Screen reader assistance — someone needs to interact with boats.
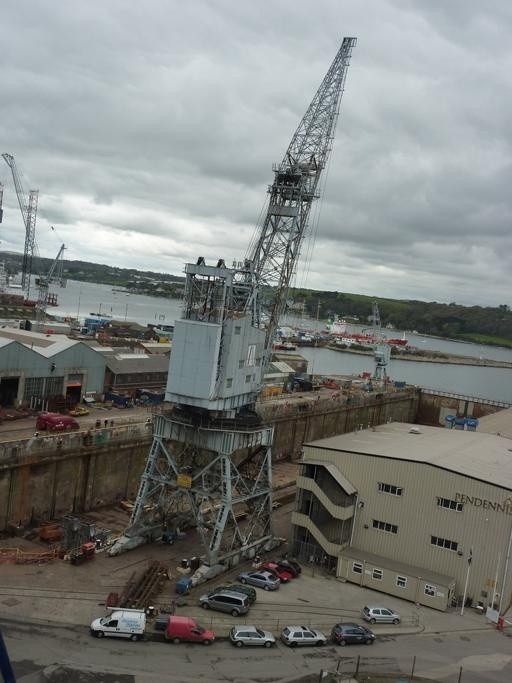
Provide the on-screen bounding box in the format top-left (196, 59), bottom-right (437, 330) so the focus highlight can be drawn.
top-left (261, 297), bottom-right (409, 354)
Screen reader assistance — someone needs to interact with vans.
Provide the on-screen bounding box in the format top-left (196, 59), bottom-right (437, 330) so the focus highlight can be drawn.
top-left (91, 606), bottom-right (147, 642)
top-left (164, 615), bottom-right (216, 646)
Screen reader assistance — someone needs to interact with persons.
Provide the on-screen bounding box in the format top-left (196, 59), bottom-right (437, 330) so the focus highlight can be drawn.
top-left (145, 415), bottom-right (152, 432)
top-left (111, 419), bottom-right (114, 427)
top-left (104, 418), bottom-right (109, 428)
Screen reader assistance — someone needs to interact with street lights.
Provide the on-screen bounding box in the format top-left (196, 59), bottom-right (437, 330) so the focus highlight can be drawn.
top-left (459, 547), bottom-right (472, 615)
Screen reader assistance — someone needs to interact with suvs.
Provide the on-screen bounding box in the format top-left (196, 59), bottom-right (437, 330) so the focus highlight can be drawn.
top-left (331, 622), bottom-right (375, 645)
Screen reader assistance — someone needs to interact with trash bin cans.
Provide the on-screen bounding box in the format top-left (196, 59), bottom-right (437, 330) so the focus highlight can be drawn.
top-left (81, 543), bottom-right (96, 561)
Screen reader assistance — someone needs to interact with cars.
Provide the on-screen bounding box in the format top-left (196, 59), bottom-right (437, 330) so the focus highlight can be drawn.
top-left (199, 556), bottom-right (302, 616)
top-left (1, 403), bottom-right (90, 433)
top-left (280, 624), bottom-right (328, 647)
top-left (155, 612), bottom-right (170, 629)
top-left (230, 625), bottom-right (276, 648)
top-left (363, 604), bottom-right (400, 625)
